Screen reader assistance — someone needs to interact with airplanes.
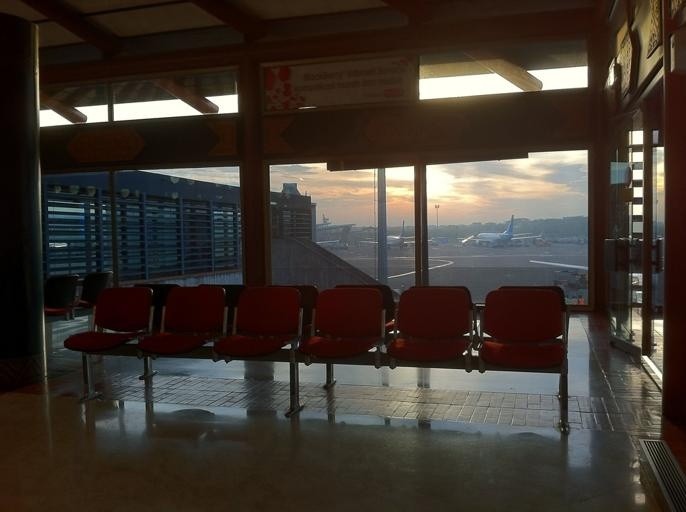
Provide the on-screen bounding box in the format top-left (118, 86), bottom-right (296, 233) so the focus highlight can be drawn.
top-left (456, 214), bottom-right (543, 247)
top-left (359, 218), bottom-right (433, 249)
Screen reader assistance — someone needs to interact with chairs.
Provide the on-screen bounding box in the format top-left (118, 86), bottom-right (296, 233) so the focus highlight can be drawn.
top-left (43, 271), bottom-right (570, 435)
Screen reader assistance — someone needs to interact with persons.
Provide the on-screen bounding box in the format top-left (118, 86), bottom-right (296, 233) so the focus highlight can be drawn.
top-left (569, 296), bottom-right (577, 305)
top-left (564, 294), bottom-right (569, 305)
top-left (577, 295), bottom-right (584, 304)
